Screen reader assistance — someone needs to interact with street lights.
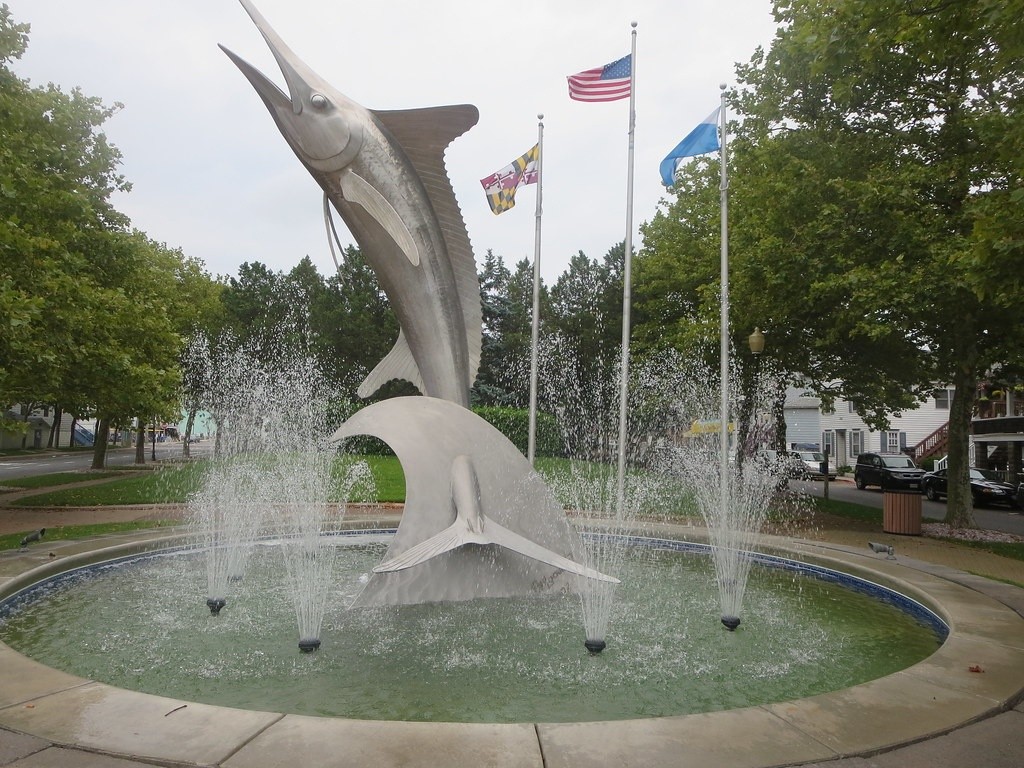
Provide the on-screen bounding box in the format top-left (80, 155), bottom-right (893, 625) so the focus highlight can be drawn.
top-left (748, 325), bottom-right (765, 461)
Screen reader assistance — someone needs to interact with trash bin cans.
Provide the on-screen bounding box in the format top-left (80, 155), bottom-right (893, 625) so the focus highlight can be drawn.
top-left (881, 471), bottom-right (925, 536)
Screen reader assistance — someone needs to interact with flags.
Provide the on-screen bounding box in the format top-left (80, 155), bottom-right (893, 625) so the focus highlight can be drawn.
top-left (659, 106), bottom-right (722, 186)
top-left (565, 53), bottom-right (631, 103)
top-left (480, 143), bottom-right (538, 215)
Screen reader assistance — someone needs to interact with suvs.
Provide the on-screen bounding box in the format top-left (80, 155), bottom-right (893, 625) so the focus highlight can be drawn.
top-left (854, 451), bottom-right (927, 493)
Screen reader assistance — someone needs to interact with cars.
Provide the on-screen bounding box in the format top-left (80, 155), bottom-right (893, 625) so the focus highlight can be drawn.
top-left (751, 450), bottom-right (838, 481)
top-left (160, 437), bottom-right (172, 442)
top-left (921, 466), bottom-right (1016, 507)
top-left (187, 433), bottom-right (200, 444)
